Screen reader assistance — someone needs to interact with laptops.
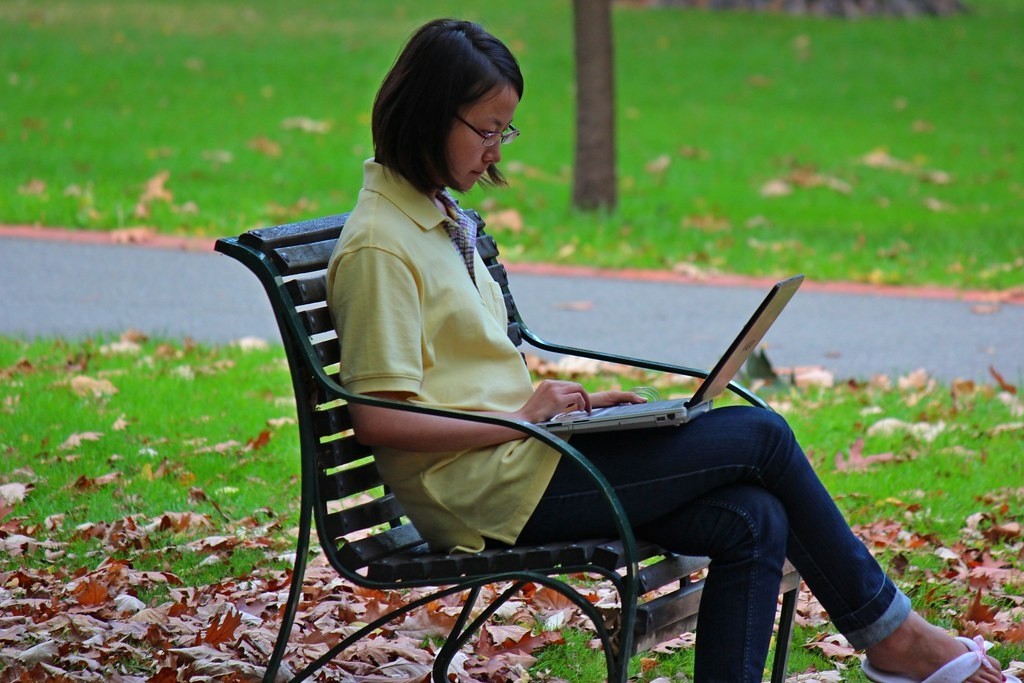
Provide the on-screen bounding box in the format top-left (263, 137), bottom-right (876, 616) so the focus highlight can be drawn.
top-left (533, 273), bottom-right (806, 432)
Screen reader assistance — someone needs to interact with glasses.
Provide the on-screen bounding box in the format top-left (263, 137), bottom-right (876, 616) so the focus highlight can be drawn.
top-left (457, 114), bottom-right (519, 147)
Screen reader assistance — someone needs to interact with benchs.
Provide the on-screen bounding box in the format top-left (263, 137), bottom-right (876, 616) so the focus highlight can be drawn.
top-left (211, 206), bottom-right (802, 683)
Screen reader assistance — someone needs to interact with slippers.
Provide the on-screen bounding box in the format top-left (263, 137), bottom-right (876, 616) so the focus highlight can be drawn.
top-left (861, 634), bottom-right (1022, 683)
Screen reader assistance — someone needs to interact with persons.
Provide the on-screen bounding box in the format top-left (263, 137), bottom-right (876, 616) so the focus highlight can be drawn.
top-left (326, 19), bottom-right (1022, 683)
